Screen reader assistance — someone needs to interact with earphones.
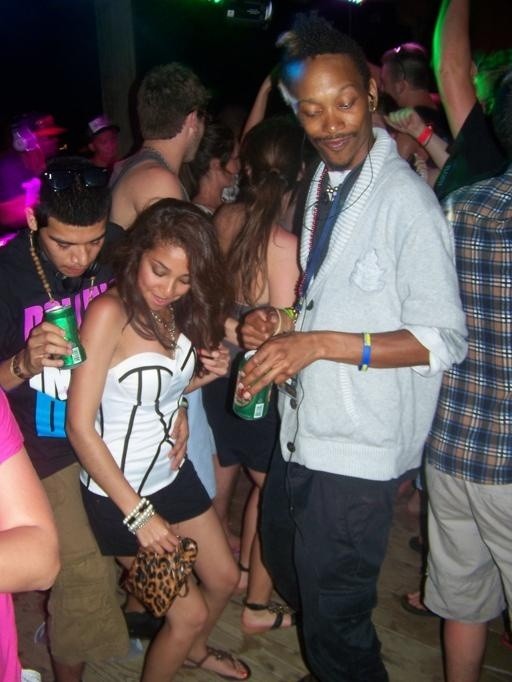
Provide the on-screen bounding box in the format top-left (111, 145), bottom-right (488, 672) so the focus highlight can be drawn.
top-left (365, 94), bottom-right (375, 107)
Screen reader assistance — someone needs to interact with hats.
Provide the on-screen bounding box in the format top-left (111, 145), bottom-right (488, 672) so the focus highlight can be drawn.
top-left (81, 114), bottom-right (120, 146)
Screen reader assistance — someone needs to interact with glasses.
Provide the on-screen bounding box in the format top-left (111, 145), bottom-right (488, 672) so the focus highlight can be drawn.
top-left (392, 45), bottom-right (406, 81)
top-left (40, 167), bottom-right (111, 192)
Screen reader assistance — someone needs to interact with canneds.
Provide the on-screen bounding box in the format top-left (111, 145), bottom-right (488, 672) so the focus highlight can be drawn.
top-left (230, 349), bottom-right (271, 421)
top-left (41, 304), bottom-right (87, 371)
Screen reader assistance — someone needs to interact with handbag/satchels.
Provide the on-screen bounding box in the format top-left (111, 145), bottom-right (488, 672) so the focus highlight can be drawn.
top-left (122, 532), bottom-right (198, 618)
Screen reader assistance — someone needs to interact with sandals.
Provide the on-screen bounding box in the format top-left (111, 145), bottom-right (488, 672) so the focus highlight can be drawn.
top-left (233, 563), bottom-right (249, 597)
top-left (181, 645), bottom-right (250, 681)
top-left (239, 598), bottom-right (296, 635)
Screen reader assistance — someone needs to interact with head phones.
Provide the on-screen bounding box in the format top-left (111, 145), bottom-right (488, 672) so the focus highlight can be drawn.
top-left (12, 126), bottom-right (39, 152)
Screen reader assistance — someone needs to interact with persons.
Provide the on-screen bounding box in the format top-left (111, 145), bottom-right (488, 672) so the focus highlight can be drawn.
top-left (0, 1), bottom-right (511, 633)
top-left (0, 159), bottom-right (189, 681)
top-left (66, 194), bottom-right (251, 682)
top-left (418, 159), bottom-right (512, 682)
top-left (236, 32), bottom-right (470, 682)
top-left (0, 386), bottom-right (63, 681)
